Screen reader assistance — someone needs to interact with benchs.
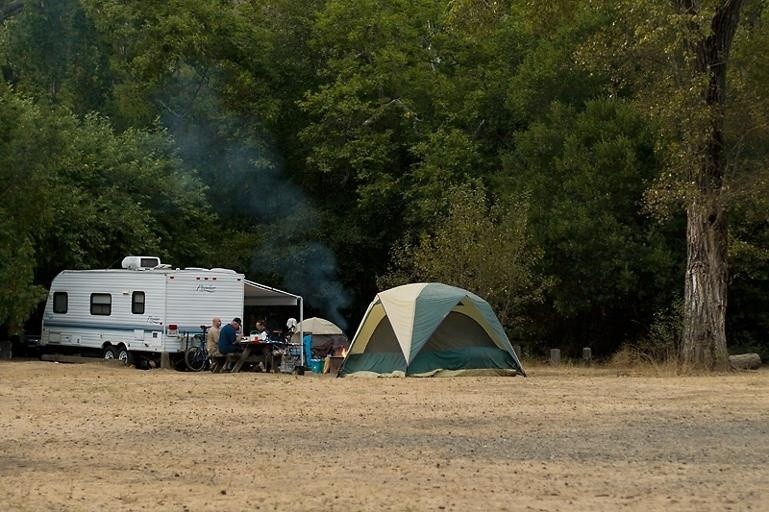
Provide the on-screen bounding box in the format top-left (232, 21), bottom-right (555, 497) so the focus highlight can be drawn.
top-left (207, 351), bottom-right (241, 373)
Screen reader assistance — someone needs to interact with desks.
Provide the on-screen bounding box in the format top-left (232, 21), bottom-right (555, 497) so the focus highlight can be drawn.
top-left (231, 340), bottom-right (301, 373)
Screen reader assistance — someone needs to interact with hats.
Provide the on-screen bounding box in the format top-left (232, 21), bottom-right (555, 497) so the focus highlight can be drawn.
top-left (233, 318), bottom-right (242, 327)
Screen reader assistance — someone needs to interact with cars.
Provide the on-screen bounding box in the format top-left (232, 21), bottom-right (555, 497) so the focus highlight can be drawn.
top-left (12, 329), bottom-right (41, 355)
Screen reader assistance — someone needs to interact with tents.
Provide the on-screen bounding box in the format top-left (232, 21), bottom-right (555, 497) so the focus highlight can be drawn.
top-left (336, 283), bottom-right (526, 378)
top-left (290, 317), bottom-right (350, 356)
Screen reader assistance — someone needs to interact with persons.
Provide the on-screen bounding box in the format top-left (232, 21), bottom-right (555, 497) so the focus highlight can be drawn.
top-left (255, 320), bottom-right (283, 341)
top-left (207, 318), bottom-right (244, 372)
top-left (286, 315), bottom-right (297, 330)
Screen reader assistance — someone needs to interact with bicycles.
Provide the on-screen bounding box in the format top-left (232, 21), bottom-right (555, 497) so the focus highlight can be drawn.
top-left (185, 336), bottom-right (213, 372)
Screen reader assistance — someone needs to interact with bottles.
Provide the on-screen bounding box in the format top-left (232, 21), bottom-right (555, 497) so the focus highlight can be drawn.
top-left (255, 333), bottom-right (258, 341)
